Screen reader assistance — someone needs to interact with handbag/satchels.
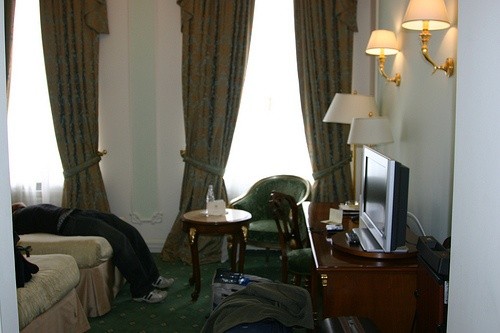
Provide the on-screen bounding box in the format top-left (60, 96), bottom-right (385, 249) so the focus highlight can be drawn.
top-left (210, 275), bottom-right (269, 316)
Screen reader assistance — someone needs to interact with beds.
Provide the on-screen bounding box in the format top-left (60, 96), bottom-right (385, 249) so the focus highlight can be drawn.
top-left (13, 233), bottom-right (128, 333)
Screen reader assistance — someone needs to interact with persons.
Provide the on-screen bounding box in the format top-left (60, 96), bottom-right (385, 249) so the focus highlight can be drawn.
top-left (11, 202), bottom-right (175, 303)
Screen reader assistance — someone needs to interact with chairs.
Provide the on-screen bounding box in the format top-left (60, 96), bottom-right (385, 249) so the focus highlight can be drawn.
top-left (269, 190), bottom-right (319, 321)
top-left (230, 176), bottom-right (307, 274)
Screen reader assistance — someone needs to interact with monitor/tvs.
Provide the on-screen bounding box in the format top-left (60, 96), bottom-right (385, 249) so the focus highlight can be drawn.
top-left (352, 145), bottom-right (410, 253)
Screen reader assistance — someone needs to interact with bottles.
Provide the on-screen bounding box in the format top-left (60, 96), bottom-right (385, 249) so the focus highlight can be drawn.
top-left (206, 185), bottom-right (215, 202)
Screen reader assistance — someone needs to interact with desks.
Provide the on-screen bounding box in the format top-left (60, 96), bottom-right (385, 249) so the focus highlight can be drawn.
top-left (180, 208), bottom-right (252, 301)
top-left (301, 201), bottom-right (424, 333)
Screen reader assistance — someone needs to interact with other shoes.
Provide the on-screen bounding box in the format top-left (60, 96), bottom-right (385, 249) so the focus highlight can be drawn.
top-left (153, 276), bottom-right (174, 288)
top-left (133, 290), bottom-right (167, 303)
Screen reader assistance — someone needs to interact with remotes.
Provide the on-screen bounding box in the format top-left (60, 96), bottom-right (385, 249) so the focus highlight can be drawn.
top-left (346, 232), bottom-right (359, 243)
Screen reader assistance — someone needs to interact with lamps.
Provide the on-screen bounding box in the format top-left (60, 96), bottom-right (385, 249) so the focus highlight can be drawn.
top-left (365, 30), bottom-right (401, 86)
top-left (401, 0), bottom-right (454, 78)
top-left (322, 90), bottom-right (394, 202)
